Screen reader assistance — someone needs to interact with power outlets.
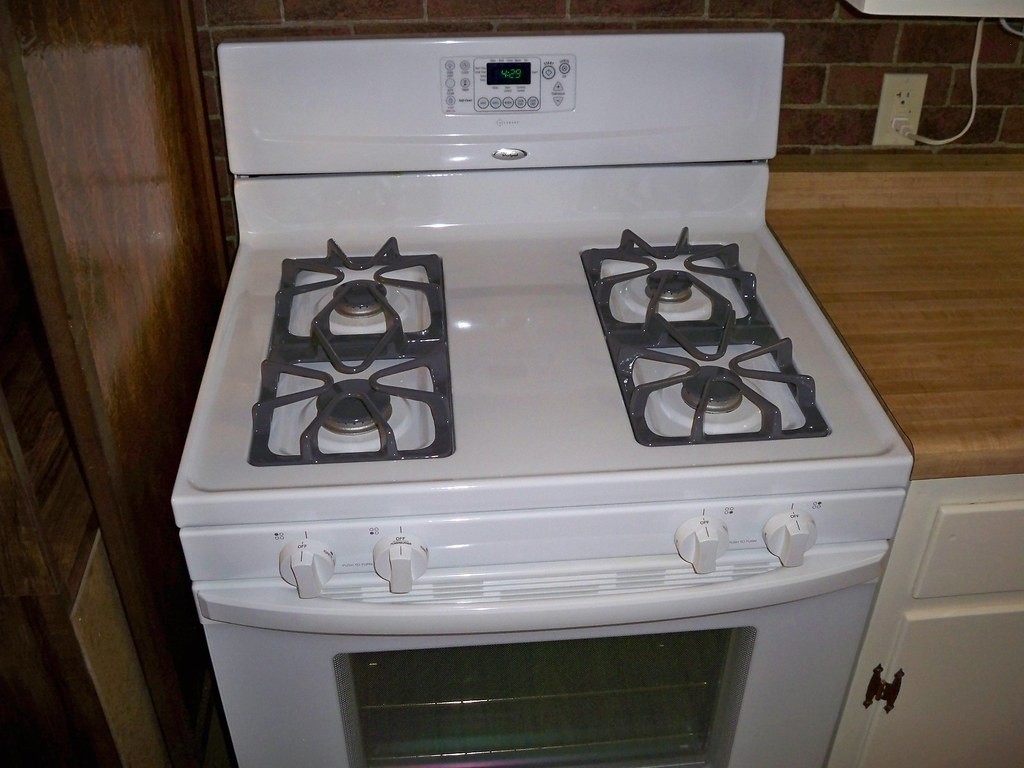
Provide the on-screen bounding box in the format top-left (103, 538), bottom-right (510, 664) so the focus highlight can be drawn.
top-left (871, 73), bottom-right (928, 148)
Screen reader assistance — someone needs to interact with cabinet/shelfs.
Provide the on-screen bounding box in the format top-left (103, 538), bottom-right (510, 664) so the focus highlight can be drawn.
top-left (764, 150), bottom-right (1024, 768)
